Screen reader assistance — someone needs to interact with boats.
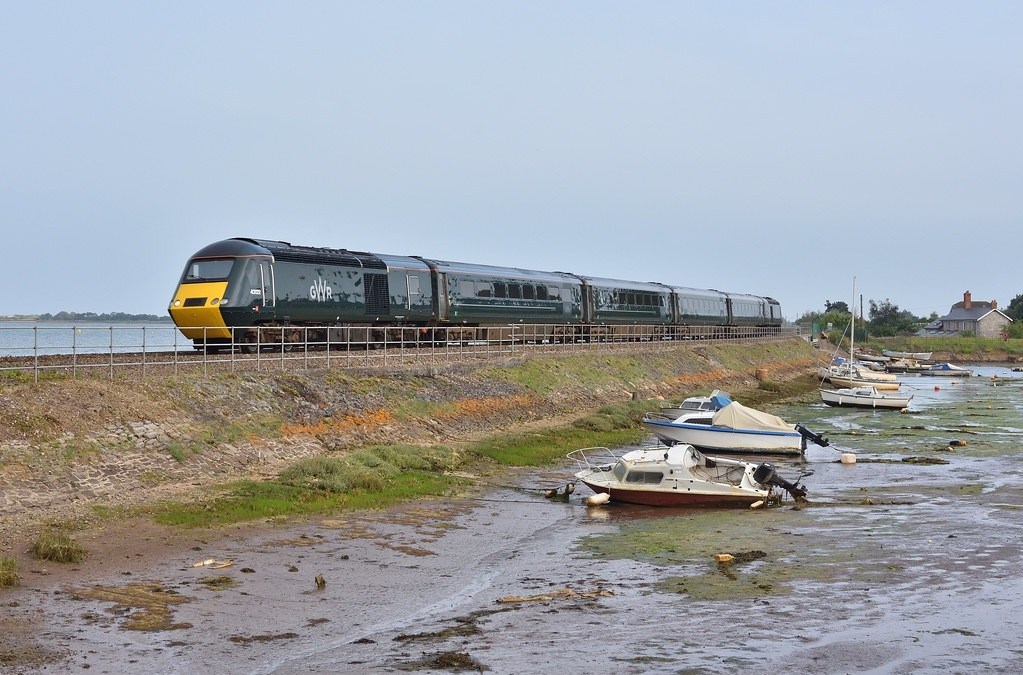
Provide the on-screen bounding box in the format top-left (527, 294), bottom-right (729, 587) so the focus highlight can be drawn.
top-left (565, 444), bottom-right (782, 506)
top-left (919, 361), bottom-right (971, 376)
top-left (659, 392), bottom-right (733, 419)
top-left (636, 403), bottom-right (810, 456)
top-left (817, 348), bottom-right (930, 390)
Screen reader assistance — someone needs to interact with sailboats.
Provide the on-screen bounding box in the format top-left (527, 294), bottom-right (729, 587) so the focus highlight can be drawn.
top-left (820, 278), bottom-right (909, 408)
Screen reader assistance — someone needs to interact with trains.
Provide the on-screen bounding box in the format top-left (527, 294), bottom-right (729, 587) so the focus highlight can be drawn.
top-left (167, 237), bottom-right (785, 352)
